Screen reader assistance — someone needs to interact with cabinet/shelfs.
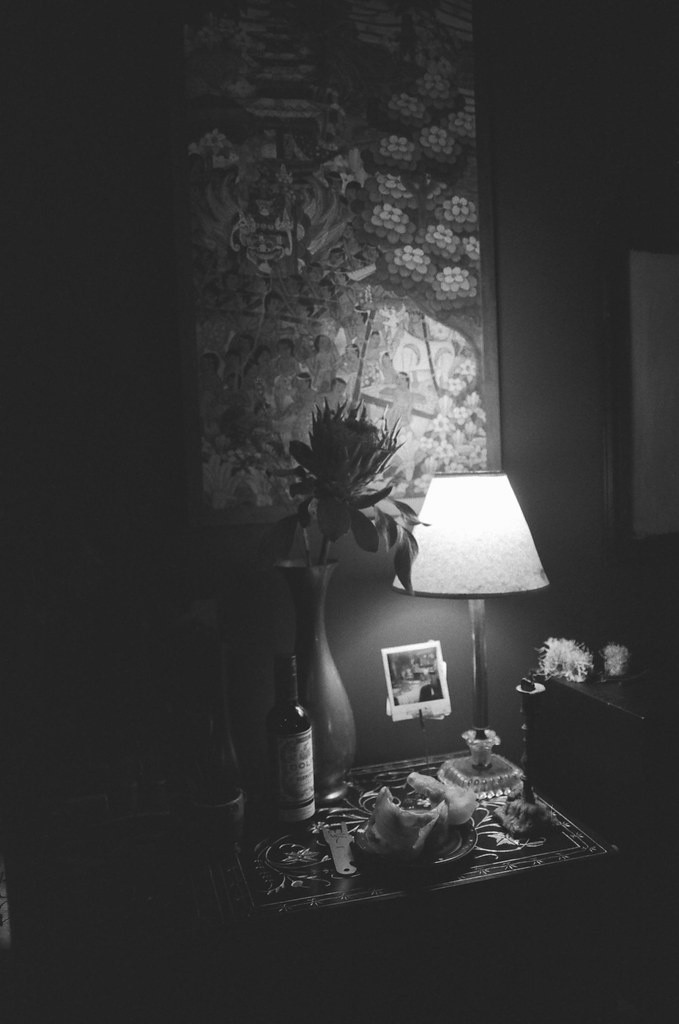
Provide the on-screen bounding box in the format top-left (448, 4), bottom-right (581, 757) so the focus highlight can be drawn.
top-left (530, 660), bottom-right (679, 1024)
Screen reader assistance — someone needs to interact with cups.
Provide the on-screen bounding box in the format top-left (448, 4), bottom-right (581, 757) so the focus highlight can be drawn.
top-left (191, 786), bottom-right (245, 838)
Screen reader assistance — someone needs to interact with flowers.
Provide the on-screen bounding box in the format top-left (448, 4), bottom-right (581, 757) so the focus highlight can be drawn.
top-left (282, 398), bottom-right (417, 600)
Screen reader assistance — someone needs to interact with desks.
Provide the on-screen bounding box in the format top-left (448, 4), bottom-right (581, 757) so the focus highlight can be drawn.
top-left (190, 752), bottom-right (621, 1024)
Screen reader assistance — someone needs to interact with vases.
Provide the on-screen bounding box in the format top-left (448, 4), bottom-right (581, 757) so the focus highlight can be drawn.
top-left (294, 556), bottom-right (358, 803)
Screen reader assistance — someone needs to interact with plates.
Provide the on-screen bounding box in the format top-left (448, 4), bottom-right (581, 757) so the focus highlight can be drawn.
top-left (355, 808), bottom-right (477, 868)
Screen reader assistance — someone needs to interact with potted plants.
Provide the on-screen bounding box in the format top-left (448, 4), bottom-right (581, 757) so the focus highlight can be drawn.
top-left (179, 704), bottom-right (247, 851)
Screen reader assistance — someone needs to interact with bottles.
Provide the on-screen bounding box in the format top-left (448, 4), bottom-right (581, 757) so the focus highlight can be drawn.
top-left (269, 655), bottom-right (316, 827)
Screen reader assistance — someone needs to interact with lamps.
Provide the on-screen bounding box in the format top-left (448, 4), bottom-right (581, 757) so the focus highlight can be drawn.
top-left (391, 469), bottom-right (552, 801)
